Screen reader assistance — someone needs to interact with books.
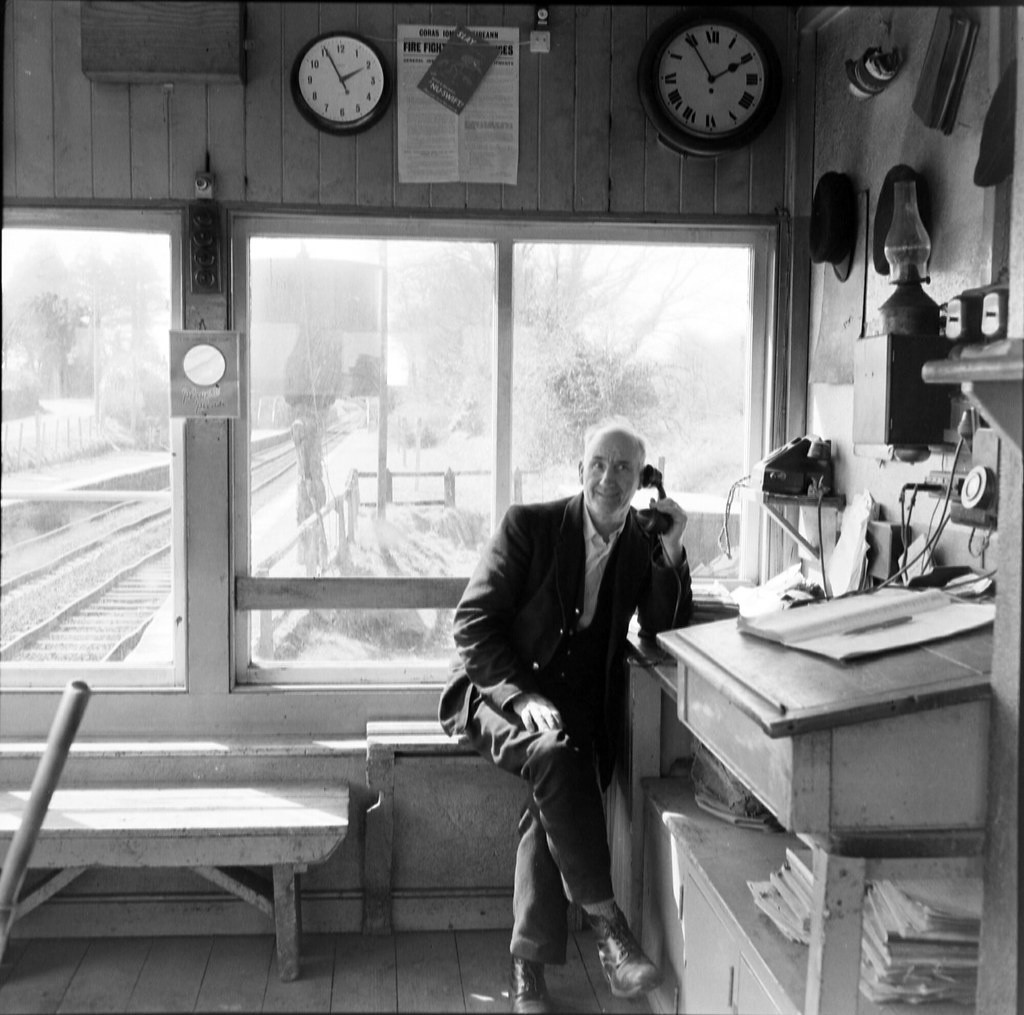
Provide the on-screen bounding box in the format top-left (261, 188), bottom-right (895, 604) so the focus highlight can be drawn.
top-left (737, 582), bottom-right (998, 663)
top-left (746, 844), bottom-right (983, 1006)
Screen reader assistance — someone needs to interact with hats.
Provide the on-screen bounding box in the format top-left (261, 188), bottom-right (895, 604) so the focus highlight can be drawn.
top-left (872, 163), bottom-right (936, 276)
top-left (806, 171), bottom-right (859, 282)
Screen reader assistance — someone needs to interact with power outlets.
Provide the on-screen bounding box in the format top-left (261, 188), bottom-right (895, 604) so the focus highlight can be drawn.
top-left (928, 470), bottom-right (952, 499)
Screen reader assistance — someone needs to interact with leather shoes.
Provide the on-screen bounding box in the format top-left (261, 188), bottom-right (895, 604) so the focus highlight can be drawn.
top-left (507, 952), bottom-right (553, 1015)
top-left (580, 900), bottom-right (660, 999)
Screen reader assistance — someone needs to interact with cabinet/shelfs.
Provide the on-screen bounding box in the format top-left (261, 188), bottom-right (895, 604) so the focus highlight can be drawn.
top-left (640, 776), bottom-right (976, 1015)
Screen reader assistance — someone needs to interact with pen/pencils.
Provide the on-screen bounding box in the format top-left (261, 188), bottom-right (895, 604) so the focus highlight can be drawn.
top-left (842, 615), bottom-right (913, 637)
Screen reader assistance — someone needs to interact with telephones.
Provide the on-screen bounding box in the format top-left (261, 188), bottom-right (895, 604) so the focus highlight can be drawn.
top-left (639, 463), bottom-right (674, 535)
top-left (753, 432), bottom-right (833, 495)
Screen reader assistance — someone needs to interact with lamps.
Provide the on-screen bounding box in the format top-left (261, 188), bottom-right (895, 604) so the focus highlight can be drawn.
top-left (877, 180), bottom-right (940, 337)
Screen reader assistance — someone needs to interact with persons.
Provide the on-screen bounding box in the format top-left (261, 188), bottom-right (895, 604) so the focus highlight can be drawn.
top-left (435, 421), bottom-right (696, 1014)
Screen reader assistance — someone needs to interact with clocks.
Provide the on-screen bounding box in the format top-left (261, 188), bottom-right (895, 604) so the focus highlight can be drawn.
top-left (290, 30), bottom-right (393, 137)
top-left (637, 8), bottom-right (783, 159)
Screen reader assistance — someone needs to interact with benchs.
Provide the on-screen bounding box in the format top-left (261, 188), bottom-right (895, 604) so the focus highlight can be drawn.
top-left (0, 782), bottom-right (350, 982)
